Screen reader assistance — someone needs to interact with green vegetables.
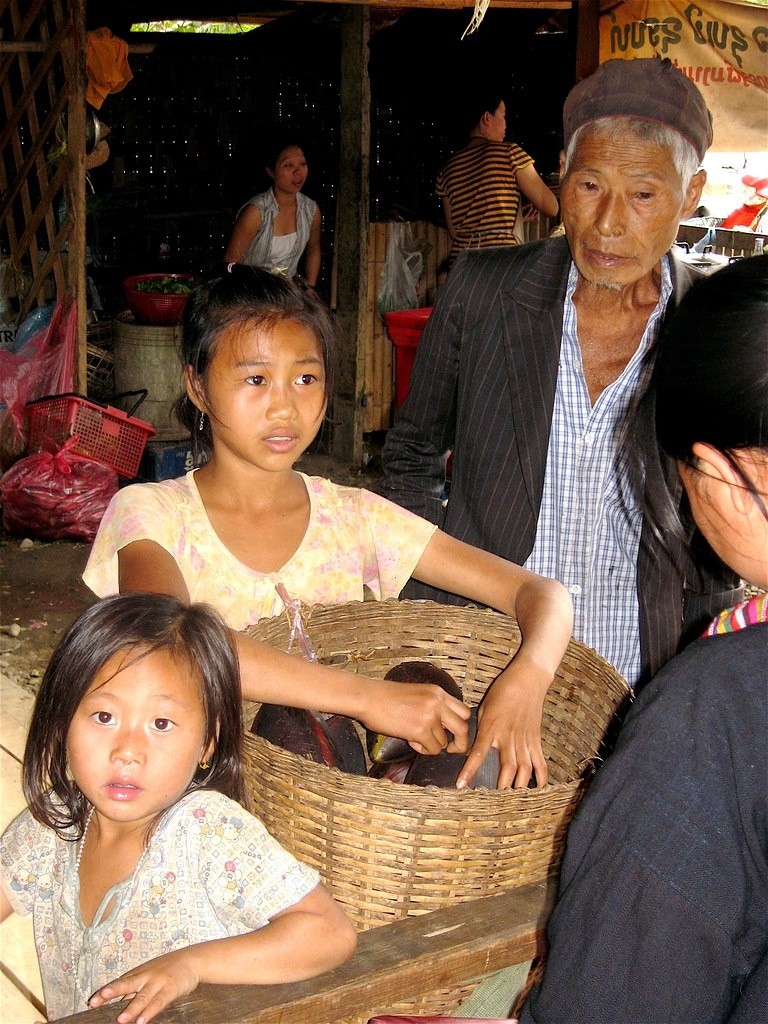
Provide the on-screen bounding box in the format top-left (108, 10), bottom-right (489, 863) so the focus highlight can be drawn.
top-left (137, 277), bottom-right (192, 294)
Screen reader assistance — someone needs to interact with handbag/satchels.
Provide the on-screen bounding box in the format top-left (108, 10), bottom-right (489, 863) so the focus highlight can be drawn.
top-left (377, 223), bottom-right (424, 325)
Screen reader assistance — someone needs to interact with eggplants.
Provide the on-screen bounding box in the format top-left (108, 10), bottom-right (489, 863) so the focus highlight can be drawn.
top-left (249, 659), bottom-right (535, 790)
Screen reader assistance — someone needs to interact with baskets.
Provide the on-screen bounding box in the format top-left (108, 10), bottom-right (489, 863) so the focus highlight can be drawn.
top-left (26, 389), bottom-right (156, 478)
top-left (122, 274), bottom-right (195, 326)
top-left (86, 319), bottom-right (119, 402)
top-left (233, 597), bottom-right (637, 1024)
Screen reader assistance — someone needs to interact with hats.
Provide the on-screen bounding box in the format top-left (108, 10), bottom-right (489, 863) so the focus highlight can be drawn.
top-left (563, 58), bottom-right (713, 167)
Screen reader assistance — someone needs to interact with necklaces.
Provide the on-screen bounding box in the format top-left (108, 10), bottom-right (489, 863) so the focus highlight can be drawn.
top-left (70, 805), bottom-right (152, 1008)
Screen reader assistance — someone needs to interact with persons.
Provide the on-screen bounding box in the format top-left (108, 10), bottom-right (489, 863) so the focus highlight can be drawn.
top-left (432, 87), bottom-right (558, 267)
top-left (224, 137), bottom-right (322, 295)
top-left (1, 588), bottom-right (359, 1020)
top-left (78, 258), bottom-right (577, 794)
top-left (375, 57), bottom-right (749, 715)
top-left (513, 252), bottom-right (767, 1024)
